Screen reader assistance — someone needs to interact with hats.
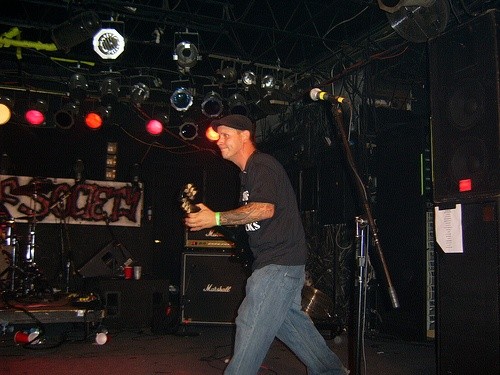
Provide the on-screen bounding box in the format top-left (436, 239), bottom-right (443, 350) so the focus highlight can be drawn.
top-left (212, 114), bottom-right (254, 131)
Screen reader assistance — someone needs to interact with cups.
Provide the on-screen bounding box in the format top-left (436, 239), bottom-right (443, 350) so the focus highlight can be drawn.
top-left (28, 333), bottom-right (39, 344)
top-left (95, 333), bottom-right (112, 345)
top-left (14, 331), bottom-right (28, 343)
top-left (133, 266), bottom-right (142, 280)
top-left (125, 267), bottom-right (133, 278)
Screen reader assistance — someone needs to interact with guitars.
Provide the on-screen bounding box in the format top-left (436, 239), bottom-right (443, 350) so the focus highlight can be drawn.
top-left (182, 184), bottom-right (253, 276)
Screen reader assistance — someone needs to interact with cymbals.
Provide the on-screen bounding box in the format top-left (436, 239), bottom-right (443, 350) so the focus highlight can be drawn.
top-left (15, 215), bottom-right (47, 220)
top-left (11, 183), bottom-right (55, 194)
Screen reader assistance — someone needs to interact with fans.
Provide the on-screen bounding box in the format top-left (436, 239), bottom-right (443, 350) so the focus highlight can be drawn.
top-left (384, 0), bottom-right (450, 44)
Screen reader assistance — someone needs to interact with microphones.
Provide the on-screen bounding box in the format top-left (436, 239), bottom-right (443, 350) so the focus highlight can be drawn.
top-left (309, 87), bottom-right (349, 103)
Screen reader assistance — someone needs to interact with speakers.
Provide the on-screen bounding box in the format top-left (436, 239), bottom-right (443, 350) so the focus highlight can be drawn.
top-left (427, 9), bottom-right (500, 202)
top-left (435, 197), bottom-right (500, 375)
top-left (179, 251), bottom-right (251, 326)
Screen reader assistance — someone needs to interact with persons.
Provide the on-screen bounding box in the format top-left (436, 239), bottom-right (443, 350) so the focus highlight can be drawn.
top-left (184, 114), bottom-right (350, 375)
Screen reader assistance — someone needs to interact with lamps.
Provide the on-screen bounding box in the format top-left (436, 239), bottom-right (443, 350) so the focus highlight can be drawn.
top-left (0, 11), bottom-right (277, 142)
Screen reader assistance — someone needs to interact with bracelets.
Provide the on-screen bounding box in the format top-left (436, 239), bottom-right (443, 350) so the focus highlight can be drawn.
top-left (216, 212), bottom-right (220, 226)
